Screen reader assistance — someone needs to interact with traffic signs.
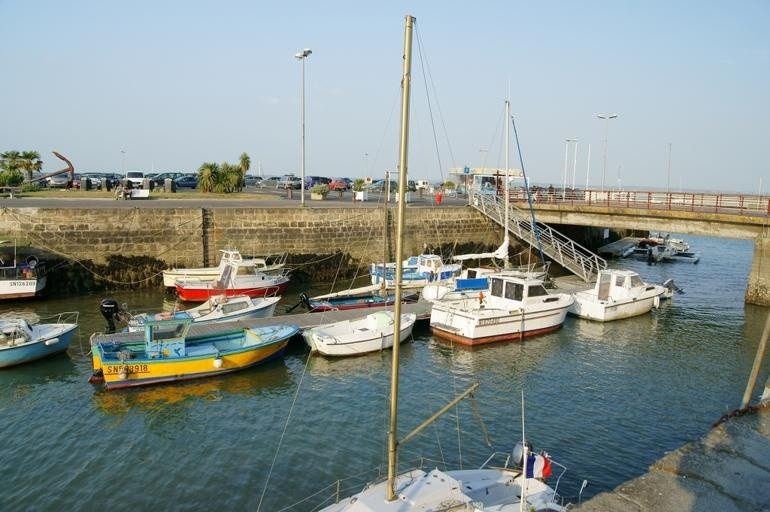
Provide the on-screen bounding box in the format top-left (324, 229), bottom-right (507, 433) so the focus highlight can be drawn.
top-left (597, 113), bottom-right (618, 192)
top-left (295, 48), bottom-right (313, 207)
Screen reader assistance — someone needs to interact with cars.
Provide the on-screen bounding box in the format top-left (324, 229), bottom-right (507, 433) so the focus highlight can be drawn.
top-left (46, 171), bottom-right (197, 188)
top-left (244, 175), bottom-right (430, 192)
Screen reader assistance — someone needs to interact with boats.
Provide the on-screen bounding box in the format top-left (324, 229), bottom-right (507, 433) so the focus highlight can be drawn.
top-left (95, 321), bottom-right (299, 391)
top-left (650, 245), bottom-right (701, 265)
top-left (419, 263), bottom-right (548, 304)
top-left (89, 302), bottom-right (437, 381)
top-left (428, 274), bottom-right (575, 345)
top-left (116, 293), bottom-right (284, 332)
top-left (272, 449), bottom-right (572, 512)
top-left (303, 310), bottom-right (416, 356)
top-left (646, 237), bottom-right (690, 251)
top-left (370, 254), bottom-right (463, 285)
top-left (0, 237), bottom-right (48, 301)
top-left (162, 248), bottom-right (287, 291)
top-left (569, 268), bottom-right (668, 321)
top-left (1, 307), bottom-right (80, 370)
top-left (298, 281), bottom-right (418, 311)
top-left (174, 259), bottom-right (289, 303)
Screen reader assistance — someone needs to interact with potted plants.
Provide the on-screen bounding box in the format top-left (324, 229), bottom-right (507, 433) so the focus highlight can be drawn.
top-left (309, 183), bottom-right (328, 201)
top-left (351, 178), bottom-right (369, 201)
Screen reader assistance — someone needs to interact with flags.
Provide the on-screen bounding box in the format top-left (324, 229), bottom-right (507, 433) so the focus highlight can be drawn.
top-left (525, 446), bottom-right (553, 481)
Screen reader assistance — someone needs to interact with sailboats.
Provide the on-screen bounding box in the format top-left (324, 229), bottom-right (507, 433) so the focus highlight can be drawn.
top-left (452, 99), bottom-right (552, 283)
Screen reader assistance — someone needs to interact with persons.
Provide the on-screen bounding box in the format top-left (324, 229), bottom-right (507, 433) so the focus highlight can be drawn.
top-left (113, 180), bottom-right (123, 201)
top-left (484, 179), bottom-right (494, 195)
top-left (124, 180), bottom-right (134, 201)
top-left (531, 185), bottom-right (539, 203)
top-left (548, 184), bottom-right (555, 201)
top-left (100, 290), bottom-right (121, 334)
